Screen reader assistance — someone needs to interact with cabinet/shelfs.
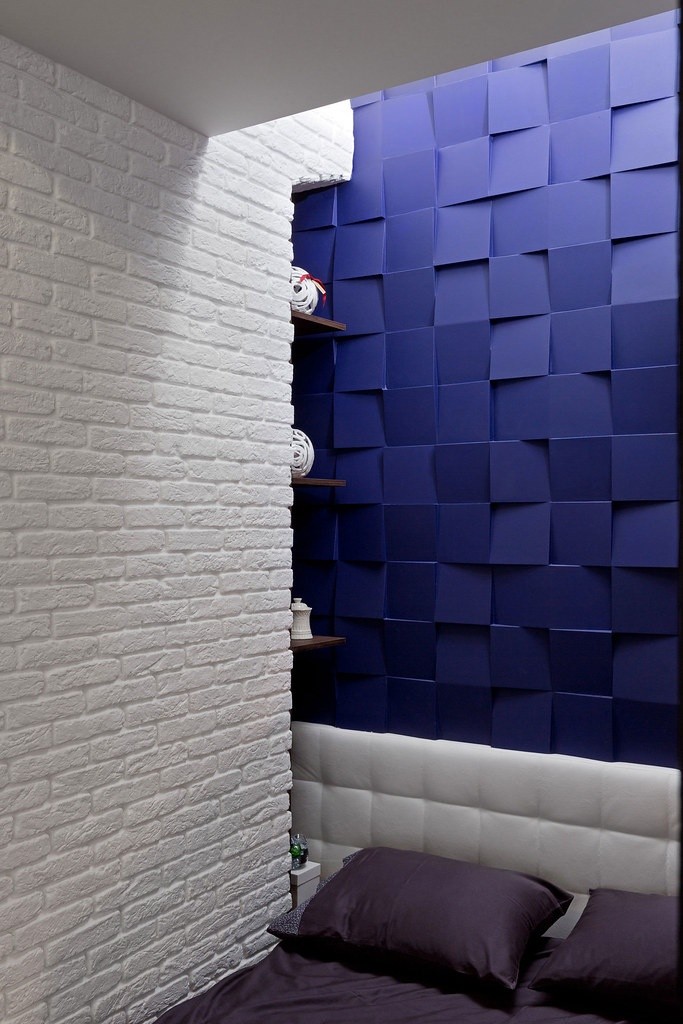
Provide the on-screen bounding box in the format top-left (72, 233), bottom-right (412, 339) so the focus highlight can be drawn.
top-left (292, 305), bottom-right (346, 650)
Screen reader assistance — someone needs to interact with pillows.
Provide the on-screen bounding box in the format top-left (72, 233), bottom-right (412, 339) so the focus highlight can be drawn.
top-left (529, 886), bottom-right (683, 1000)
top-left (264, 845), bottom-right (574, 993)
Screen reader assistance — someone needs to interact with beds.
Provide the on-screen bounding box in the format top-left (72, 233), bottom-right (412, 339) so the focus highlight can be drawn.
top-left (150, 720), bottom-right (683, 1024)
